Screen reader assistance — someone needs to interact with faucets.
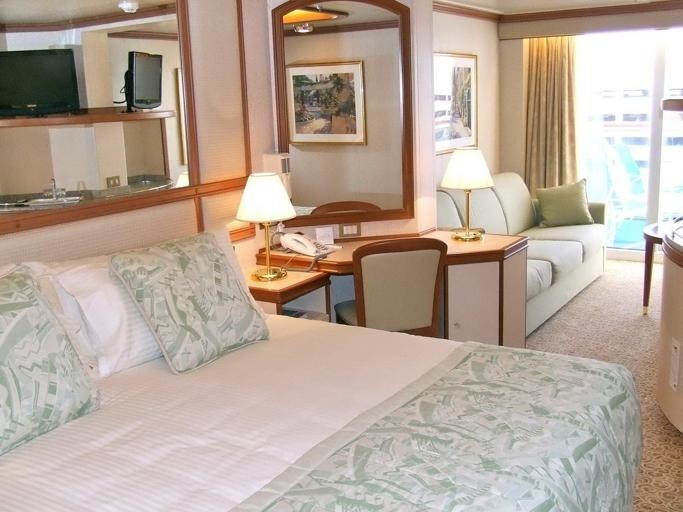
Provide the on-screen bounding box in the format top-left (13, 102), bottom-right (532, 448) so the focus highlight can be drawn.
top-left (49, 177), bottom-right (58, 200)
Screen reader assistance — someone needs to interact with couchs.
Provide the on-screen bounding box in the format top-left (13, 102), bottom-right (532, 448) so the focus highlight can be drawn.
top-left (435, 171), bottom-right (608, 337)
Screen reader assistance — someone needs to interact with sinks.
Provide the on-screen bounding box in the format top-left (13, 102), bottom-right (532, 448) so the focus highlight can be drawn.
top-left (25, 196), bottom-right (82, 206)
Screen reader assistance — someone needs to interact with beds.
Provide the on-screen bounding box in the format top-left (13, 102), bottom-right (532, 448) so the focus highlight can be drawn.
top-left (0, 314), bottom-right (636, 512)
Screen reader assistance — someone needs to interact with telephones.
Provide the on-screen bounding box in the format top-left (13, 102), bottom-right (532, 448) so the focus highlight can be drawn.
top-left (280, 232), bottom-right (331, 256)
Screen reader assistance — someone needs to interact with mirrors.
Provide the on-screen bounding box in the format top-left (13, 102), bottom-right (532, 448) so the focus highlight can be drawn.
top-left (0, 0), bottom-right (200, 226)
top-left (272, 0), bottom-right (418, 228)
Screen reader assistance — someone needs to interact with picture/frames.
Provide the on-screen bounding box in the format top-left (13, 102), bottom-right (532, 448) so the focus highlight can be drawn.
top-left (286, 59), bottom-right (368, 148)
top-left (433, 51), bottom-right (481, 157)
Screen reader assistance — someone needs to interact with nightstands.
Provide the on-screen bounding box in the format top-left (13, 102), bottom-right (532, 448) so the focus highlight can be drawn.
top-left (245, 265), bottom-right (330, 330)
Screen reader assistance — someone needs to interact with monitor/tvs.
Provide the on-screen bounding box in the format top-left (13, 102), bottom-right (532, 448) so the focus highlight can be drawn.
top-left (122, 51), bottom-right (163, 113)
top-left (0, 49), bottom-right (81, 119)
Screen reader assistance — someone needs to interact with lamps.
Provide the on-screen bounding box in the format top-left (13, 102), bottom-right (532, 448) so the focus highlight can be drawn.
top-left (234, 171), bottom-right (294, 284)
top-left (442, 148), bottom-right (496, 243)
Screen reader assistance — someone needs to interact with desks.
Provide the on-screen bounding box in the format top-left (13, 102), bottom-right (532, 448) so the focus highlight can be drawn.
top-left (255, 232), bottom-right (529, 350)
top-left (640, 222), bottom-right (670, 315)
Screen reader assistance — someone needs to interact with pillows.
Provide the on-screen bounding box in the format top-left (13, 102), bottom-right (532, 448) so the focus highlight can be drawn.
top-left (534, 178), bottom-right (596, 228)
